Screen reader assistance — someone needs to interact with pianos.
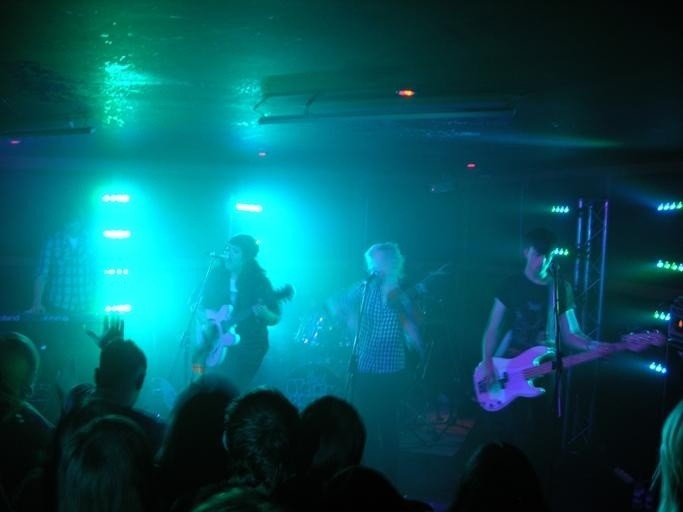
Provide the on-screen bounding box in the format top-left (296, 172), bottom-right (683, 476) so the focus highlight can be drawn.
top-left (0, 307), bottom-right (106, 327)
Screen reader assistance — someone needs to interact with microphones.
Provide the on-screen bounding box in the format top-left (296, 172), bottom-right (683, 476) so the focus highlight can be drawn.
top-left (208, 252), bottom-right (230, 259)
top-left (360, 271), bottom-right (378, 287)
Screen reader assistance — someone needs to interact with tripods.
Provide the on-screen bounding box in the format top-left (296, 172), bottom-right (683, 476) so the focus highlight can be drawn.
top-left (418, 297), bottom-right (472, 441)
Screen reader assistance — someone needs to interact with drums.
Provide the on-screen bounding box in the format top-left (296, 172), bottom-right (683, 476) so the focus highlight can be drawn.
top-left (293, 314), bottom-right (341, 349)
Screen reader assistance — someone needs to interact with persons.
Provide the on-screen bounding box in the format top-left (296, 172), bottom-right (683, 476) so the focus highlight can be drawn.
top-left (1, 319), bottom-right (431, 512)
top-left (651, 400), bottom-right (683, 512)
top-left (330, 240), bottom-right (423, 424)
top-left (192, 234), bottom-right (282, 391)
top-left (446, 440), bottom-right (547, 512)
top-left (478, 227), bottom-right (616, 426)
top-left (18, 200), bottom-right (108, 374)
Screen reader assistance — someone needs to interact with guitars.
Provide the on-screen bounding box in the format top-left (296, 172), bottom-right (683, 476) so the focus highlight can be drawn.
top-left (473, 330), bottom-right (666, 412)
top-left (195, 285), bottom-right (293, 369)
